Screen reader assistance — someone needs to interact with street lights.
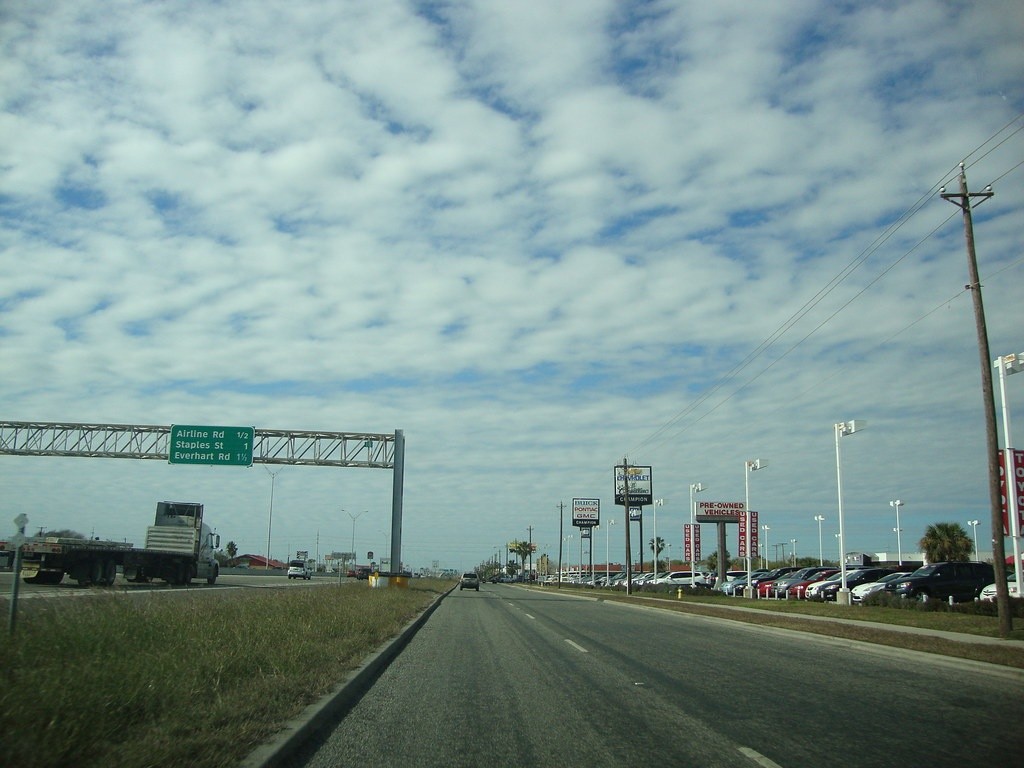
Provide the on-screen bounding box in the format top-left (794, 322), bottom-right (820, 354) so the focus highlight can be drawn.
top-left (262, 461), bottom-right (289, 570)
top-left (592, 525), bottom-right (602, 583)
top-left (653, 499), bottom-right (671, 585)
top-left (341, 508), bottom-right (369, 569)
top-left (814, 515), bottom-right (825, 567)
top-left (689, 482), bottom-right (708, 586)
top-left (790, 538), bottom-right (797, 568)
top-left (606, 519), bottom-right (618, 585)
top-left (993, 350), bottom-right (1024, 599)
top-left (967, 519), bottom-right (981, 562)
top-left (890, 499), bottom-right (905, 566)
top-left (578, 529), bottom-right (589, 583)
top-left (833, 419), bottom-right (868, 593)
top-left (744, 458), bottom-right (769, 589)
top-left (762, 525), bottom-right (771, 570)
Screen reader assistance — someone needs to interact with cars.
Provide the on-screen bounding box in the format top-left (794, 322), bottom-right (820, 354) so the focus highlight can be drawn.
top-left (346, 570), bottom-right (431, 581)
top-left (541, 560), bottom-right (1024, 604)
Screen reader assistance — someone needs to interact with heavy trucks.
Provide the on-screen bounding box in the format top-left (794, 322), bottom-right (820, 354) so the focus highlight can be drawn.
top-left (0, 501), bottom-right (222, 587)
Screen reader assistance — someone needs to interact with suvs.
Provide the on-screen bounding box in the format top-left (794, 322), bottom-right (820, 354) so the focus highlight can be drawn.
top-left (458, 573), bottom-right (481, 591)
top-left (287, 560), bottom-right (313, 580)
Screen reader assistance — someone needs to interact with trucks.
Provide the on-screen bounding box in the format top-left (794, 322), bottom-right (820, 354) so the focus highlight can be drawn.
top-left (379, 557), bottom-right (404, 577)
